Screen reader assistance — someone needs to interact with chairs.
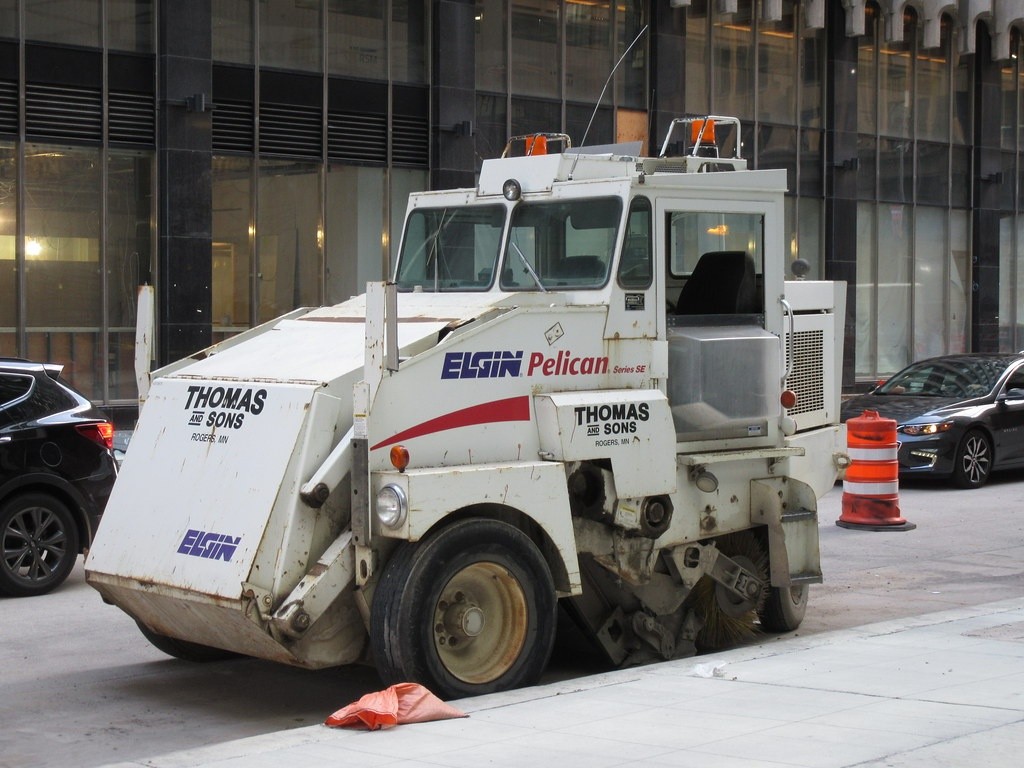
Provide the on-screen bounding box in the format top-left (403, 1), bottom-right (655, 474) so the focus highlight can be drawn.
top-left (549, 255), bottom-right (608, 281)
top-left (676, 251), bottom-right (756, 314)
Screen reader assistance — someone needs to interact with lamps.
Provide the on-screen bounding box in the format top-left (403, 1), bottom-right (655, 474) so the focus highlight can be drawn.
top-left (187, 92), bottom-right (207, 113)
top-left (843, 158), bottom-right (860, 170)
top-left (670, 140), bottom-right (685, 156)
top-left (989, 172), bottom-right (1005, 184)
top-left (459, 121), bottom-right (473, 138)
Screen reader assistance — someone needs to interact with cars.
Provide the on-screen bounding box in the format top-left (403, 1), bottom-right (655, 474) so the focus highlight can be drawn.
top-left (840, 351), bottom-right (1024, 490)
top-left (0, 356), bottom-right (119, 594)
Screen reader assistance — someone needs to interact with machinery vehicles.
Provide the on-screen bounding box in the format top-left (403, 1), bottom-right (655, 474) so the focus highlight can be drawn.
top-left (85, 24), bottom-right (848, 701)
